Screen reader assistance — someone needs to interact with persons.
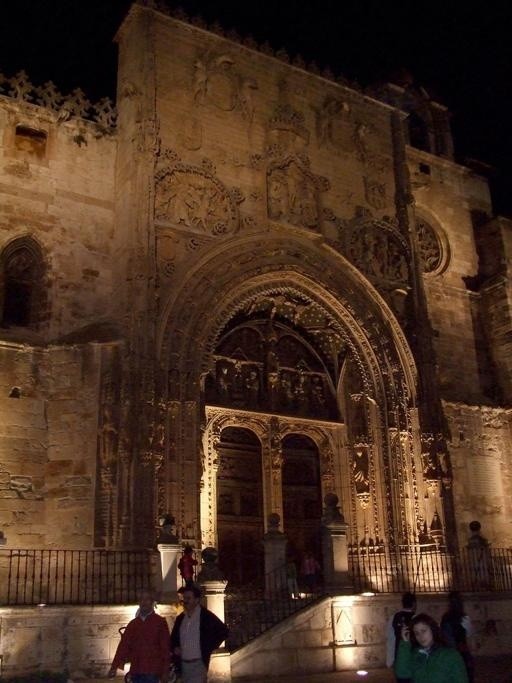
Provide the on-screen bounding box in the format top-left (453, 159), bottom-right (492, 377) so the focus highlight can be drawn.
top-left (219, 339), bottom-right (325, 410)
top-left (170, 586), bottom-right (229, 682)
top-left (393, 612), bottom-right (468, 683)
top-left (439, 593), bottom-right (475, 682)
top-left (286, 558), bottom-right (299, 594)
top-left (177, 546), bottom-right (198, 585)
top-left (109, 591), bottom-right (171, 682)
top-left (384, 591), bottom-right (416, 682)
top-left (299, 553), bottom-right (321, 588)
